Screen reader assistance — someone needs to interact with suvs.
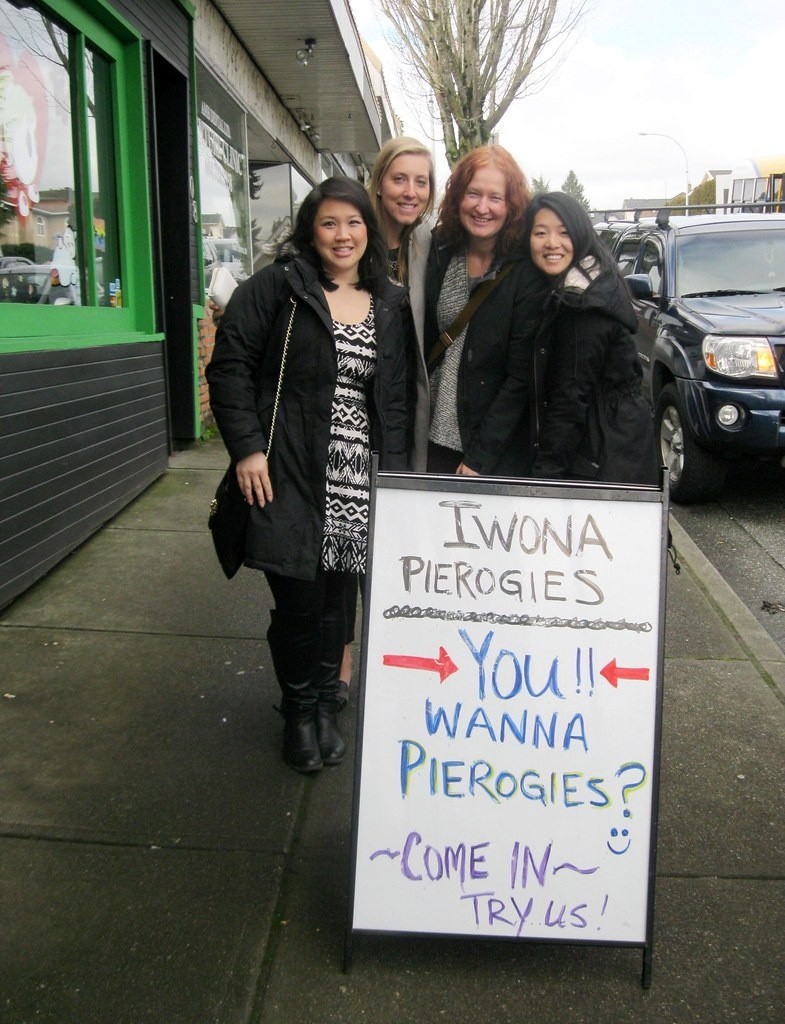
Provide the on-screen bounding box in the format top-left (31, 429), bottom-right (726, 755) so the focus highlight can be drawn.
top-left (589, 208), bottom-right (785, 509)
top-left (0, 255), bottom-right (105, 308)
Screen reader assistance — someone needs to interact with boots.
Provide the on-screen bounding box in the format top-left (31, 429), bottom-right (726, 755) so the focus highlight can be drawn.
top-left (266, 609), bottom-right (323, 773)
top-left (315, 611), bottom-right (347, 764)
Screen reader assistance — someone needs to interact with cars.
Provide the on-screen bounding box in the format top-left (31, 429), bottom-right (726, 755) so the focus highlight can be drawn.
top-left (202, 235), bottom-right (244, 289)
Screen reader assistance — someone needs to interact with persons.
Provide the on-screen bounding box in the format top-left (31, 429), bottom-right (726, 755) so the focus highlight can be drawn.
top-left (204, 137), bottom-right (654, 776)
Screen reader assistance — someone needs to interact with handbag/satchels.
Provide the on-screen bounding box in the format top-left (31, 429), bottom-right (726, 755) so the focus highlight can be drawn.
top-left (207, 461), bottom-right (250, 580)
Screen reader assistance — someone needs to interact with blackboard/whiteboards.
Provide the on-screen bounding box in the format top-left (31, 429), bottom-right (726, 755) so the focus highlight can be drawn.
top-left (343, 450), bottom-right (672, 950)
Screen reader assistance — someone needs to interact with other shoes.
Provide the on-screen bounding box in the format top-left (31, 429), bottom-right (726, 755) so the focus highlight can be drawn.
top-left (334, 681), bottom-right (348, 712)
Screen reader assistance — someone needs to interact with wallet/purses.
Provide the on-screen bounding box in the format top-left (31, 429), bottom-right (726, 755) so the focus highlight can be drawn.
top-left (208, 267), bottom-right (239, 311)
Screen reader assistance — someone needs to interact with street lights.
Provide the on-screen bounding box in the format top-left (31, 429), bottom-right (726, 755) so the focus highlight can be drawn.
top-left (641, 132), bottom-right (689, 216)
